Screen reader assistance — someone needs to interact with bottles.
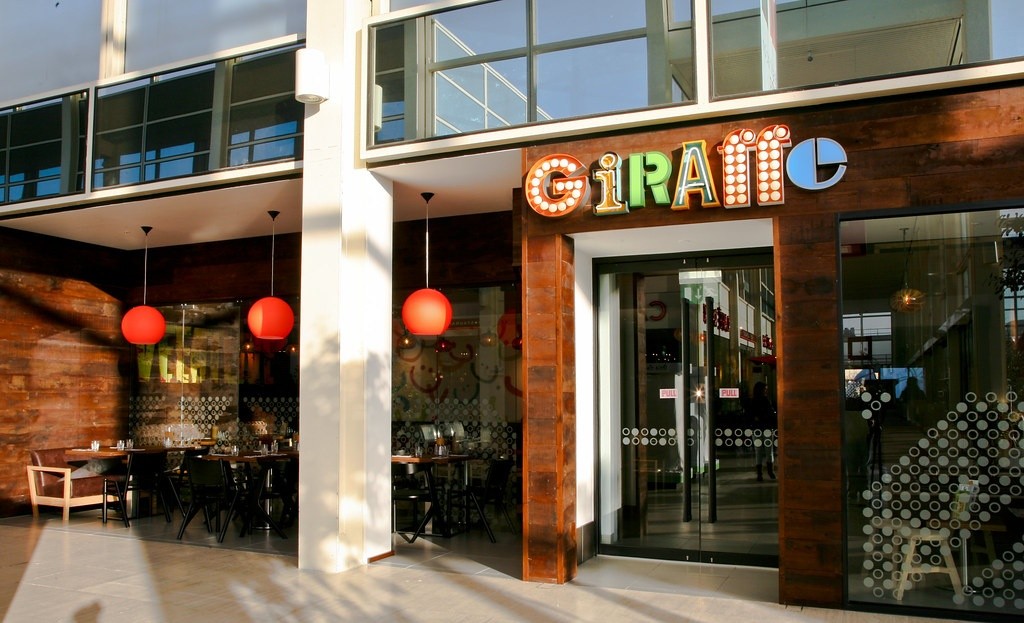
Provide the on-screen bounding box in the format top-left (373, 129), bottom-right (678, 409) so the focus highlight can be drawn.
top-left (434, 438), bottom-right (447, 456)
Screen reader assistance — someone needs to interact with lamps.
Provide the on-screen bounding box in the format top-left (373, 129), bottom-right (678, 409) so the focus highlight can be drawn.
top-left (888, 228), bottom-right (927, 313)
top-left (402, 192), bottom-right (454, 335)
top-left (121, 226), bottom-right (167, 346)
top-left (247, 210), bottom-right (296, 341)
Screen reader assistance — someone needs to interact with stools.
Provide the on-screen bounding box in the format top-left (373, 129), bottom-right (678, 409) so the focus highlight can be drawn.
top-left (896, 530), bottom-right (965, 600)
top-left (861, 518), bottom-right (926, 592)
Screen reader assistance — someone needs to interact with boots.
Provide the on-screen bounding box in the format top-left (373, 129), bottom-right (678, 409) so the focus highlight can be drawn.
top-left (766, 462), bottom-right (776, 479)
top-left (756, 464), bottom-right (763, 480)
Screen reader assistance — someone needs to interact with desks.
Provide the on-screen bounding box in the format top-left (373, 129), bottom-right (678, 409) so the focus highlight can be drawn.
top-left (66, 447), bottom-right (195, 521)
top-left (926, 522), bottom-right (1007, 593)
top-left (201, 451), bottom-right (300, 543)
top-left (392, 454), bottom-right (476, 543)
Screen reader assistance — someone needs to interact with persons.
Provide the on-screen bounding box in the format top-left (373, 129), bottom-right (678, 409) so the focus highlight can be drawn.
top-left (747, 381), bottom-right (778, 482)
top-left (846, 397), bottom-right (875, 505)
top-left (900, 376), bottom-right (928, 430)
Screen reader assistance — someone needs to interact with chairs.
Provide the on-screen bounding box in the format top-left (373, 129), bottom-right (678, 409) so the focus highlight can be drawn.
top-left (102, 450), bottom-right (516, 544)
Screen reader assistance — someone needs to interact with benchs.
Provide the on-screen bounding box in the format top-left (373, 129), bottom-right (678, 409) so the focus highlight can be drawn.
top-left (25, 447), bottom-right (126, 522)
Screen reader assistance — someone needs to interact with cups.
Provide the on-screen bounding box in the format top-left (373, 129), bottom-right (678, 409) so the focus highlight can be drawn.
top-left (271, 443), bottom-right (278, 454)
top-left (231, 446), bottom-right (239, 456)
top-left (127, 439), bottom-right (133, 449)
top-left (117, 440), bottom-right (125, 451)
top-left (91, 441), bottom-right (100, 452)
top-left (260, 444), bottom-right (268, 455)
top-left (164, 427), bottom-right (173, 448)
top-left (415, 444), bottom-right (422, 458)
